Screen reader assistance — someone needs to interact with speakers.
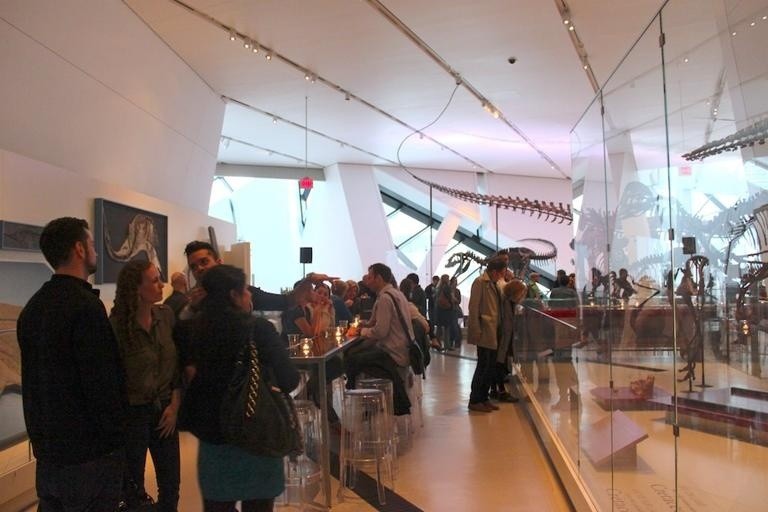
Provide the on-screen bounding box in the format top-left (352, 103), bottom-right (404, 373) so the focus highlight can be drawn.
top-left (682, 237), bottom-right (695, 254)
top-left (300, 246), bottom-right (312, 263)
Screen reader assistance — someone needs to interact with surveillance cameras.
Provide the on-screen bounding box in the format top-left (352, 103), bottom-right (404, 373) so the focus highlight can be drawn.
top-left (508, 57), bottom-right (516, 64)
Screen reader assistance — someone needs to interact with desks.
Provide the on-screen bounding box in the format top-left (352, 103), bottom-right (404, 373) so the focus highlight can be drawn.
top-left (285, 333), bottom-right (360, 508)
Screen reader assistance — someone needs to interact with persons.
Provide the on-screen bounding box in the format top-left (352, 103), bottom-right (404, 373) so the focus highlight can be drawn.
top-left (163, 271), bottom-right (191, 321)
top-left (388, 271), bottom-right (462, 354)
top-left (16, 217), bottom-right (131, 512)
top-left (109, 259), bottom-right (183, 512)
top-left (179, 264), bottom-right (300, 511)
top-left (575, 268), bottom-right (632, 353)
top-left (283, 274), bottom-right (377, 435)
top-left (467, 251), bottom-right (582, 411)
top-left (176, 240), bottom-right (340, 348)
top-left (307, 263), bottom-right (431, 435)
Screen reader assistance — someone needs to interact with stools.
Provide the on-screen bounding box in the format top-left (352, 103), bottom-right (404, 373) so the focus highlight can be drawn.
top-left (356, 378), bottom-right (398, 475)
top-left (290, 399), bottom-right (321, 488)
top-left (339, 389), bottom-right (394, 505)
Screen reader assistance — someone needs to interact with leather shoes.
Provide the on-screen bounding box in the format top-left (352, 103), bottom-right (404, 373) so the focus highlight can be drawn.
top-left (468, 389), bottom-right (518, 413)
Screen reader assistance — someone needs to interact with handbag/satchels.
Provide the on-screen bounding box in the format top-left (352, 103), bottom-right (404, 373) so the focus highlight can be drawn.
top-left (218, 361), bottom-right (303, 459)
top-left (438, 290), bottom-right (452, 310)
top-left (411, 342), bottom-right (424, 376)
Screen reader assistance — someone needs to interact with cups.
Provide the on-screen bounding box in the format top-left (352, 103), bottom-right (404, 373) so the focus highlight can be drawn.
top-left (324, 315), bottom-right (360, 338)
top-left (287, 332), bottom-right (300, 350)
top-left (300, 338), bottom-right (313, 350)
